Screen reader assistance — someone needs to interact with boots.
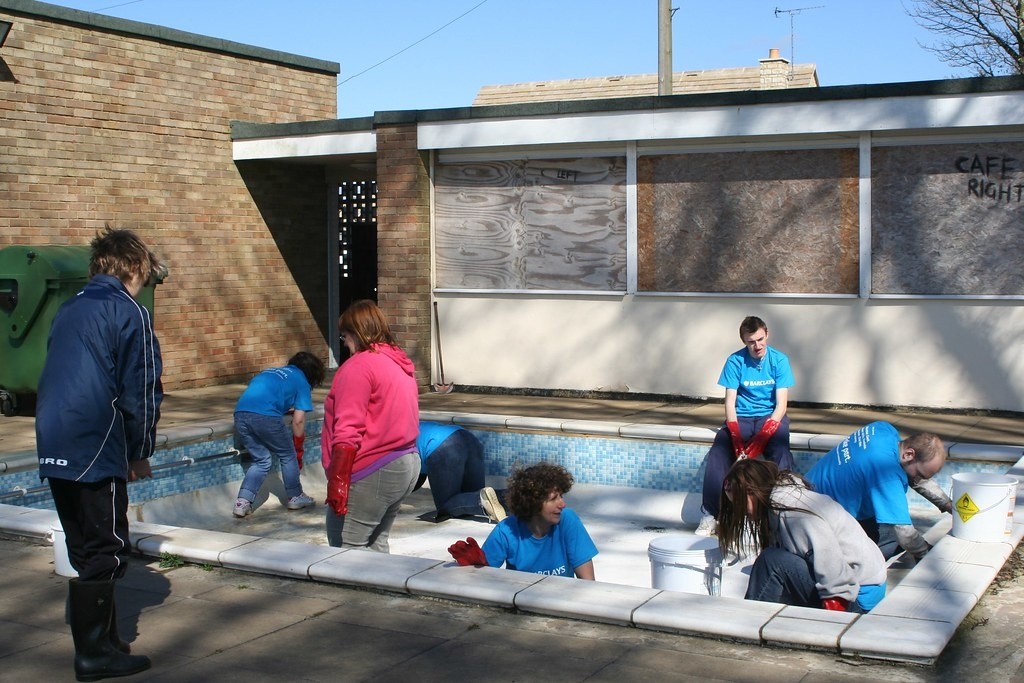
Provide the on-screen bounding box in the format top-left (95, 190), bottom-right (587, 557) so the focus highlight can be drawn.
top-left (69, 577), bottom-right (151, 682)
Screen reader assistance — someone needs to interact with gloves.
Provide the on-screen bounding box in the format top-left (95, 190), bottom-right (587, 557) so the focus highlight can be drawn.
top-left (447, 537), bottom-right (490, 567)
top-left (292, 432), bottom-right (305, 470)
top-left (726, 420), bottom-right (745, 457)
top-left (324, 444), bottom-right (358, 517)
top-left (822, 596), bottom-right (851, 612)
top-left (744, 417), bottom-right (782, 459)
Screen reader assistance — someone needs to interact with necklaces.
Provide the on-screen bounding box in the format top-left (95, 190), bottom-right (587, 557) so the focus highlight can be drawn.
top-left (751, 357), bottom-right (765, 370)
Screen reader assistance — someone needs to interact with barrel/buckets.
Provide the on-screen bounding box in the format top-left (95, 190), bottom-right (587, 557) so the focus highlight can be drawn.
top-left (50, 522), bottom-right (80, 579)
top-left (950, 473), bottom-right (1019, 543)
top-left (648, 534), bottom-right (724, 598)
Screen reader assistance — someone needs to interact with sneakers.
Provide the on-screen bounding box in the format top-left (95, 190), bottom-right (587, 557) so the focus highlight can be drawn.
top-left (287, 491), bottom-right (316, 510)
top-left (694, 512), bottom-right (719, 538)
top-left (479, 487), bottom-right (506, 523)
top-left (233, 498), bottom-right (253, 517)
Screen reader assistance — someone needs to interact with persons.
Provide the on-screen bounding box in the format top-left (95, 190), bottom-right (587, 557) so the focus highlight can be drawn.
top-left (233, 350), bottom-right (323, 517)
top-left (695, 315), bottom-right (954, 614)
top-left (35, 224), bottom-right (166, 682)
top-left (447, 462), bottom-right (599, 582)
top-left (320, 301), bottom-right (507, 553)
